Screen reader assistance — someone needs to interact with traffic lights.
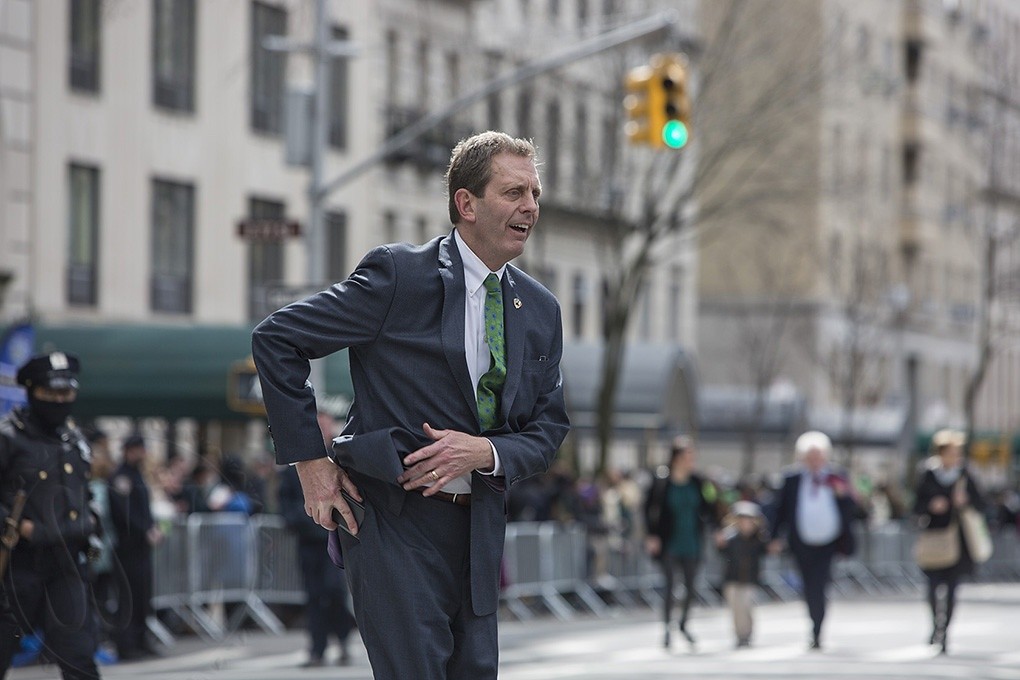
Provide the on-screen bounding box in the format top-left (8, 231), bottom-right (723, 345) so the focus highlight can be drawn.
top-left (656, 62), bottom-right (691, 149)
top-left (616, 63), bottom-right (669, 156)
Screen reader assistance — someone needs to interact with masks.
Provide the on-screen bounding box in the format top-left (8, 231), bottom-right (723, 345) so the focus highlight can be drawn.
top-left (34, 396), bottom-right (77, 423)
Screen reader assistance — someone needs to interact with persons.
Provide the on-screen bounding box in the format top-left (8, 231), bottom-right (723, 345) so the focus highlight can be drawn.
top-left (277, 412), bottom-right (358, 667)
top-left (643, 436), bottom-right (713, 646)
top-left (505, 460), bottom-right (624, 521)
top-left (250, 131), bottom-right (571, 680)
top-left (914, 428), bottom-right (981, 653)
top-left (87, 435), bottom-right (162, 662)
top-left (158, 447), bottom-right (265, 631)
top-left (717, 502), bottom-right (766, 648)
top-left (0, 352), bottom-right (100, 680)
top-left (770, 432), bottom-right (857, 651)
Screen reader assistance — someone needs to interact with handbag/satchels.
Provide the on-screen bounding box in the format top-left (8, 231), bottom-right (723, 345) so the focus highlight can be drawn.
top-left (961, 506), bottom-right (994, 565)
top-left (916, 525), bottom-right (961, 571)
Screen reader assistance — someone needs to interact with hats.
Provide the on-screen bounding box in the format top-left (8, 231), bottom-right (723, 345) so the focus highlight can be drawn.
top-left (16, 352), bottom-right (80, 391)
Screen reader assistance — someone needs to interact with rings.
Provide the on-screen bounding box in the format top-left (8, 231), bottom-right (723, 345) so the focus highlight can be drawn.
top-left (431, 471), bottom-right (439, 479)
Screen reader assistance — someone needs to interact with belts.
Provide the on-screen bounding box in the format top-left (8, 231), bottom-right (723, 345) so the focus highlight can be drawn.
top-left (414, 484), bottom-right (474, 508)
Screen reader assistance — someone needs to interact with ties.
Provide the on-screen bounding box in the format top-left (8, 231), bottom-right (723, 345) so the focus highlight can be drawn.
top-left (475, 271), bottom-right (507, 433)
